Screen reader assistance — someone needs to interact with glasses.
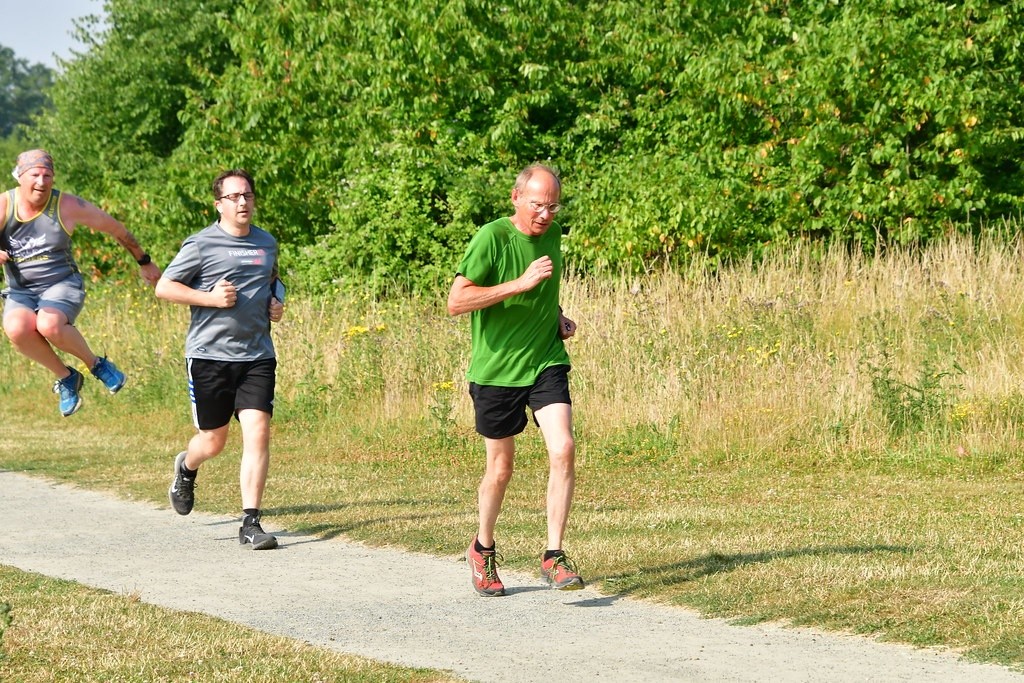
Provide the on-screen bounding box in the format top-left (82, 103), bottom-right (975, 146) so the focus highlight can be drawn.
top-left (217, 191), bottom-right (256, 201)
top-left (530, 202), bottom-right (562, 214)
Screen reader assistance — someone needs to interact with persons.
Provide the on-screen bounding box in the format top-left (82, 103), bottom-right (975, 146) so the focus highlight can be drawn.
top-left (447, 161), bottom-right (585, 597)
top-left (154, 169), bottom-right (285, 548)
top-left (0, 148), bottom-right (162, 418)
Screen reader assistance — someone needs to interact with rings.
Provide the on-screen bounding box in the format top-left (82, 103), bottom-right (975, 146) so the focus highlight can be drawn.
top-left (276, 314), bottom-right (278, 318)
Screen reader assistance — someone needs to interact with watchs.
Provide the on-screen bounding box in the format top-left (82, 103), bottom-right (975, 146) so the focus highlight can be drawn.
top-left (137, 254), bottom-right (151, 266)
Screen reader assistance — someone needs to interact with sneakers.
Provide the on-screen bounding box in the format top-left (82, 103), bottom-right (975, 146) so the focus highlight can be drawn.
top-left (168, 450), bottom-right (199, 516)
top-left (465, 533), bottom-right (507, 597)
top-left (52, 365), bottom-right (85, 417)
top-left (90, 352), bottom-right (127, 396)
top-left (541, 552), bottom-right (586, 589)
top-left (238, 510), bottom-right (278, 550)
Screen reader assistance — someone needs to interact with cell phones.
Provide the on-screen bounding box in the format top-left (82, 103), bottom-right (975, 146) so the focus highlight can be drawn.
top-left (275, 279), bottom-right (285, 303)
